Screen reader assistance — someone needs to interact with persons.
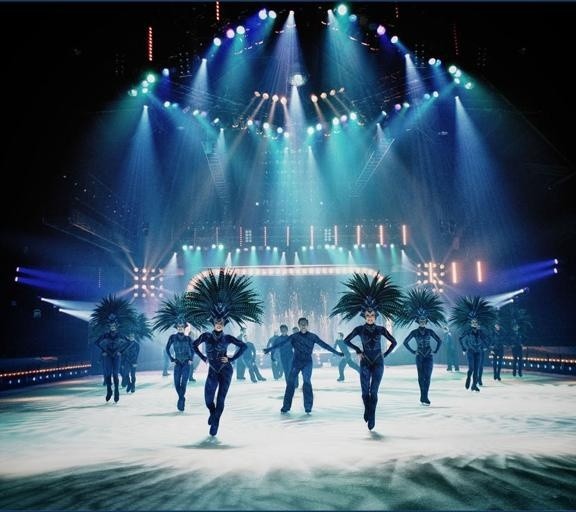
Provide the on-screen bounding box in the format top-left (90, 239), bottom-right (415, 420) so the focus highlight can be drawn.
top-left (332, 332), bottom-right (360, 381)
top-left (161, 344), bottom-right (172, 377)
top-left (262, 317), bottom-right (345, 414)
top-left (266, 325), bottom-right (300, 388)
top-left (458, 317), bottom-right (524, 391)
top-left (186, 331), bottom-right (202, 381)
top-left (192, 303), bottom-right (247, 437)
top-left (95, 319), bottom-right (140, 403)
top-left (166, 317), bottom-right (194, 411)
top-left (403, 314), bottom-right (442, 405)
top-left (235, 326), bottom-right (247, 380)
top-left (343, 305), bottom-right (398, 431)
top-left (231, 334), bottom-right (266, 383)
top-left (443, 326), bottom-right (460, 372)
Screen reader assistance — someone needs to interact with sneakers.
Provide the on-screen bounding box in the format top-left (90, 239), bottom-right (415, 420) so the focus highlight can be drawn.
top-left (207, 414), bottom-right (214, 424)
top-left (419, 392), bottom-right (431, 407)
top-left (471, 386), bottom-right (480, 392)
top-left (105, 392), bottom-right (112, 402)
top-left (209, 423), bottom-right (219, 438)
top-left (479, 381), bottom-right (483, 386)
top-left (131, 384), bottom-right (136, 393)
top-left (368, 420), bottom-right (375, 431)
top-left (177, 397), bottom-right (186, 411)
top-left (304, 406), bottom-right (313, 415)
top-left (464, 383), bottom-right (469, 390)
top-left (126, 384), bottom-right (131, 392)
top-left (363, 412), bottom-right (370, 424)
top-left (114, 393), bottom-right (120, 403)
top-left (280, 404), bottom-right (291, 414)
